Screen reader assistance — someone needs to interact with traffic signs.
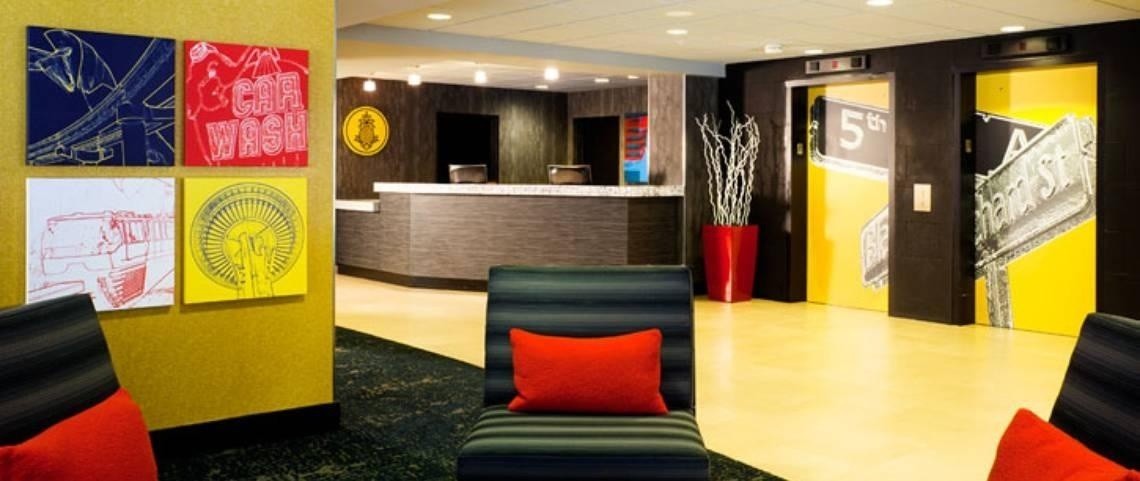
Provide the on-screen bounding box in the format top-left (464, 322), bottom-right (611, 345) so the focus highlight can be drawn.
top-left (859, 112), bottom-right (1096, 296)
top-left (810, 95), bottom-right (1044, 193)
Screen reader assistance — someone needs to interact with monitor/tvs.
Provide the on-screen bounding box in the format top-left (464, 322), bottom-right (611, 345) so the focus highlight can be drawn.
top-left (448, 163), bottom-right (487, 183)
top-left (546, 163), bottom-right (591, 184)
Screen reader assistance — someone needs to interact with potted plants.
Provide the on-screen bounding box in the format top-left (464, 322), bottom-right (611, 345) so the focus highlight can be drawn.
top-left (695, 105), bottom-right (759, 303)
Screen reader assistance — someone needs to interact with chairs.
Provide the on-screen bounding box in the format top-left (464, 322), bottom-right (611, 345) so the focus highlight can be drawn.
top-left (456, 263), bottom-right (711, 481)
top-left (447, 161), bottom-right (593, 185)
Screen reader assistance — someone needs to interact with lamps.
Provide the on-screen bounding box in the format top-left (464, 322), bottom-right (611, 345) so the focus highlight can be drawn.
top-left (362, 63), bottom-right (561, 96)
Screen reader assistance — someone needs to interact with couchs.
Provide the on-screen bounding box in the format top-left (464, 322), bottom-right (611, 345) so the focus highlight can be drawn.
top-left (1, 291), bottom-right (157, 480)
top-left (989, 311), bottom-right (1140, 481)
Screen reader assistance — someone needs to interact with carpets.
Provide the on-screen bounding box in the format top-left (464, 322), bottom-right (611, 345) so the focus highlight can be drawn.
top-left (155, 320), bottom-right (790, 481)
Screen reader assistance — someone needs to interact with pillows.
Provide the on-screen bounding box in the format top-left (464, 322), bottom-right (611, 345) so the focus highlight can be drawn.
top-left (987, 409), bottom-right (1140, 481)
top-left (506, 326), bottom-right (669, 416)
top-left (1, 379), bottom-right (158, 480)
